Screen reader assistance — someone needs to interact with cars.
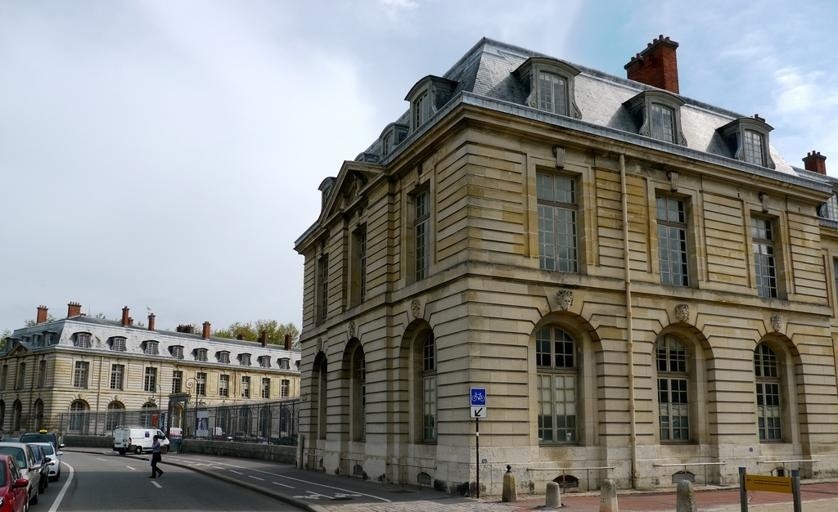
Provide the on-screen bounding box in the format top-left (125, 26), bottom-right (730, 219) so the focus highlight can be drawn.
top-left (0, 429), bottom-right (65, 512)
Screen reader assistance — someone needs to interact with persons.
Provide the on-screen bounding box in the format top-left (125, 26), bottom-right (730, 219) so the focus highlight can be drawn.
top-left (147, 434), bottom-right (163, 478)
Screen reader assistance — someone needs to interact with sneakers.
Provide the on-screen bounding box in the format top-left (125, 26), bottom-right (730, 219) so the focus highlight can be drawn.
top-left (147, 471), bottom-right (165, 479)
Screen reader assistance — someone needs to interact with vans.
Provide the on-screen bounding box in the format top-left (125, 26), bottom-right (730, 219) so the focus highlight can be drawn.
top-left (112, 424), bottom-right (170, 456)
